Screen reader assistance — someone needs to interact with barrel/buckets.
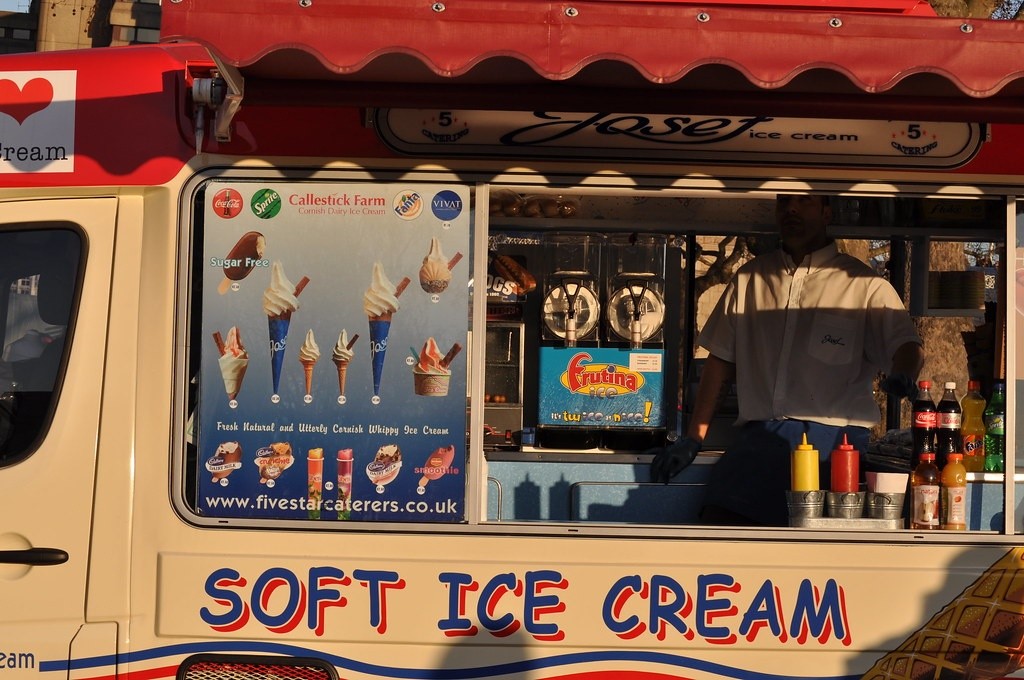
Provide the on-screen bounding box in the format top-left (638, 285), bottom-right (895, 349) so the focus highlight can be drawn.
top-left (864, 493), bottom-right (907, 519)
top-left (827, 490), bottom-right (867, 518)
top-left (786, 488), bottom-right (825, 517)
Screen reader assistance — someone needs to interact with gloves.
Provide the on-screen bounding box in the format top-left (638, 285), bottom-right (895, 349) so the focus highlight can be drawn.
top-left (650, 436), bottom-right (703, 484)
top-left (878, 369), bottom-right (919, 404)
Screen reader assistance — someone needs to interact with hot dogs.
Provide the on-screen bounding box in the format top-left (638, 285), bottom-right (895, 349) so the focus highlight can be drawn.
top-left (493, 254), bottom-right (536, 296)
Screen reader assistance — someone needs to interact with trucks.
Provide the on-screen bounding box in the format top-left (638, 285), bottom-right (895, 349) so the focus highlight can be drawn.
top-left (0, 0), bottom-right (1024, 680)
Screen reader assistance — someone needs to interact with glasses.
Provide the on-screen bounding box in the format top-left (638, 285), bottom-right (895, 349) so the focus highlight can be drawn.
top-left (776, 195), bottom-right (824, 209)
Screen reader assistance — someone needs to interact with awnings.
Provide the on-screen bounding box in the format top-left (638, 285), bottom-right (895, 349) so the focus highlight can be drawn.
top-left (162, 2), bottom-right (1022, 127)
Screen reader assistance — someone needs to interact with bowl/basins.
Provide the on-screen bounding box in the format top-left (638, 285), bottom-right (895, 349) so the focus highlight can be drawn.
top-left (865, 470), bottom-right (909, 492)
top-left (412, 371), bottom-right (451, 397)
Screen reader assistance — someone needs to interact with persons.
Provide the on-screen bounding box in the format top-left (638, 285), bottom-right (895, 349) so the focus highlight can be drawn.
top-left (651, 194), bottom-right (925, 529)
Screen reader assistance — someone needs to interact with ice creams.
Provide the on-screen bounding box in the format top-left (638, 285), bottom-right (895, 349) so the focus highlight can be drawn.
top-left (213, 324), bottom-right (248, 402)
top-left (419, 236), bottom-right (462, 293)
top-left (409, 337), bottom-right (462, 396)
top-left (258, 259), bottom-right (411, 396)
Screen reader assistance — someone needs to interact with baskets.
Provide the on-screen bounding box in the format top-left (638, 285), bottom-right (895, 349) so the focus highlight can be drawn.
top-left (928, 271), bottom-right (985, 309)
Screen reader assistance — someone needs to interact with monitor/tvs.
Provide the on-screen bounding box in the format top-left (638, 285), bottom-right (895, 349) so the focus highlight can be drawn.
top-left (542, 339), bottom-right (666, 450)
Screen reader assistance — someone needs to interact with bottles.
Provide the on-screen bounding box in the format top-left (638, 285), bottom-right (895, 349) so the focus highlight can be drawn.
top-left (910, 452), bottom-right (941, 532)
top-left (913, 381), bottom-right (937, 467)
top-left (828, 434), bottom-right (860, 493)
top-left (960, 377), bottom-right (986, 473)
top-left (939, 452), bottom-right (966, 530)
top-left (935, 382), bottom-right (962, 470)
top-left (789, 432), bottom-right (821, 492)
top-left (983, 379), bottom-right (1006, 473)
